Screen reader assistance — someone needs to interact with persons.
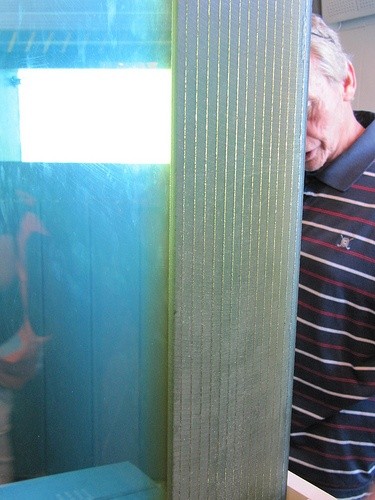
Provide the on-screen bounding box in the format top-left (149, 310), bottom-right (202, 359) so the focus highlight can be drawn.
top-left (288, 11), bottom-right (375, 500)
